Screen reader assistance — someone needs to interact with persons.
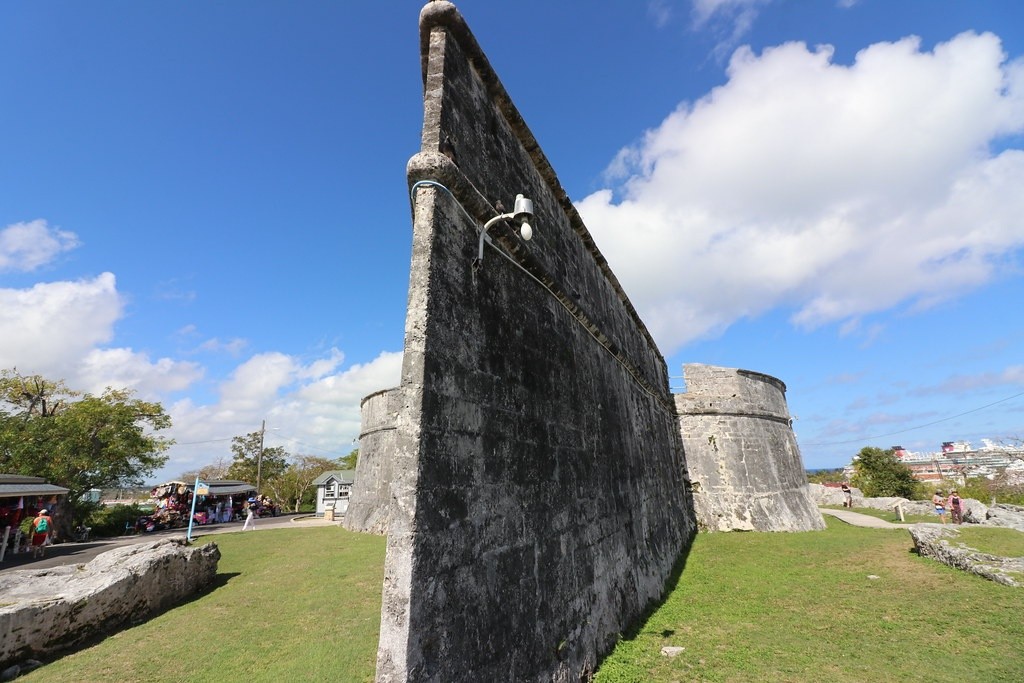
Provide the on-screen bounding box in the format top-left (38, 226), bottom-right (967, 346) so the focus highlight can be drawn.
top-left (841, 480), bottom-right (852, 507)
top-left (240, 497), bottom-right (256, 531)
top-left (28, 509), bottom-right (54, 561)
top-left (931, 487), bottom-right (964, 523)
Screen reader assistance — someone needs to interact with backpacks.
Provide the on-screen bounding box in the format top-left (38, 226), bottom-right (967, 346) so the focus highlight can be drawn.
top-left (36, 516), bottom-right (49, 534)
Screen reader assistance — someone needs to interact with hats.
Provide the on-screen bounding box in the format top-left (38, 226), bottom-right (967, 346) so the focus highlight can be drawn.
top-left (952, 490), bottom-right (957, 493)
top-left (38, 509), bottom-right (48, 514)
top-left (935, 490), bottom-right (942, 494)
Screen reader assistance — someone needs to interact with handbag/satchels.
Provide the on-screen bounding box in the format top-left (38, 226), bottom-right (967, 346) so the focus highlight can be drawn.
top-left (249, 503), bottom-right (259, 510)
top-left (935, 505), bottom-right (943, 510)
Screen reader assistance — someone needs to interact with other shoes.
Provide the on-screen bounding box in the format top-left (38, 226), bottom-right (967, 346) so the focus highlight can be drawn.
top-left (41, 554), bottom-right (45, 557)
top-left (33, 555), bottom-right (36, 558)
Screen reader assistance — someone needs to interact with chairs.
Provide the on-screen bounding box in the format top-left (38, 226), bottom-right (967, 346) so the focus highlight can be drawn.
top-left (124, 522), bottom-right (135, 535)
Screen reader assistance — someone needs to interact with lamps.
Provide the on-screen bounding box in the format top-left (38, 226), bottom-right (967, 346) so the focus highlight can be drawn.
top-left (788, 415), bottom-right (799, 428)
top-left (478, 194), bottom-right (534, 264)
